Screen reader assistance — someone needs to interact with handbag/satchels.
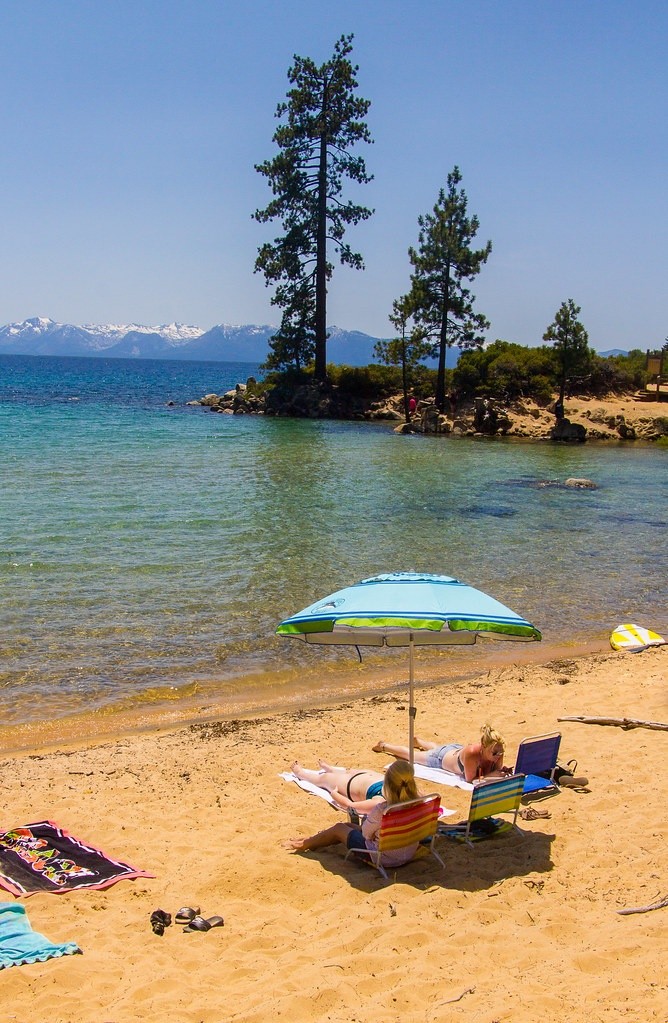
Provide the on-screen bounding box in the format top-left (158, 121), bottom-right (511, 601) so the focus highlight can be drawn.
top-left (551, 757), bottom-right (578, 786)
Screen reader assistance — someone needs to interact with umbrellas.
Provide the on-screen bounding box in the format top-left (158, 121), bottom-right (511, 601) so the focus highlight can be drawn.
top-left (275, 568), bottom-right (542, 764)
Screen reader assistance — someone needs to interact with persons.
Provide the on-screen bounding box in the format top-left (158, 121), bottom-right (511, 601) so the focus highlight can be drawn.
top-left (451, 389), bottom-right (458, 406)
top-left (409, 396), bottom-right (416, 413)
top-left (290, 760), bottom-right (419, 868)
top-left (431, 395), bottom-right (435, 405)
top-left (555, 400), bottom-right (562, 419)
top-left (372, 724), bottom-right (514, 783)
top-left (290, 759), bottom-right (387, 815)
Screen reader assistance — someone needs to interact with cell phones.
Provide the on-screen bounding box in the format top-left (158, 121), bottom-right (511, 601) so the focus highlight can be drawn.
top-left (506, 766), bottom-right (514, 773)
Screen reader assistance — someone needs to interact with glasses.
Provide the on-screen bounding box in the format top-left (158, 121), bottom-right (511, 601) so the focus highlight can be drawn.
top-left (491, 751), bottom-right (505, 756)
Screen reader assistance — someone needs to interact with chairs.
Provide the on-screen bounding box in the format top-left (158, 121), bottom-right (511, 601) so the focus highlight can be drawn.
top-left (437, 774), bottom-right (525, 848)
top-left (345, 793), bottom-right (446, 880)
top-left (514, 731), bottom-right (562, 803)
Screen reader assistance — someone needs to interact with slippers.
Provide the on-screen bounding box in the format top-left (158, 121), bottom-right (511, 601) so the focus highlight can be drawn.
top-left (183, 915), bottom-right (224, 933)
top-left (175, 906), bottom-right (200, 924)
top-left (520, 806), bottom-right (549, 820)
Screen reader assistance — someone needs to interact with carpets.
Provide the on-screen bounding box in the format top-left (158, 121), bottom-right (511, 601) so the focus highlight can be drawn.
top-left (383, 760), bottom-right (486, 790)
top-left (277, 766), bottom-right (457, 820)
top-left (0, 819), bottom-right (157, 897)
top-left (0, 902), bottom-right (83, 971)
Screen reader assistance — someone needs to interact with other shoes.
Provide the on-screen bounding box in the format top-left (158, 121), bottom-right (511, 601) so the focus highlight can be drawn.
top-left (566, 783), bottom-right (592, 793)
top-left (559, 776), bottom-right (588, 786)
